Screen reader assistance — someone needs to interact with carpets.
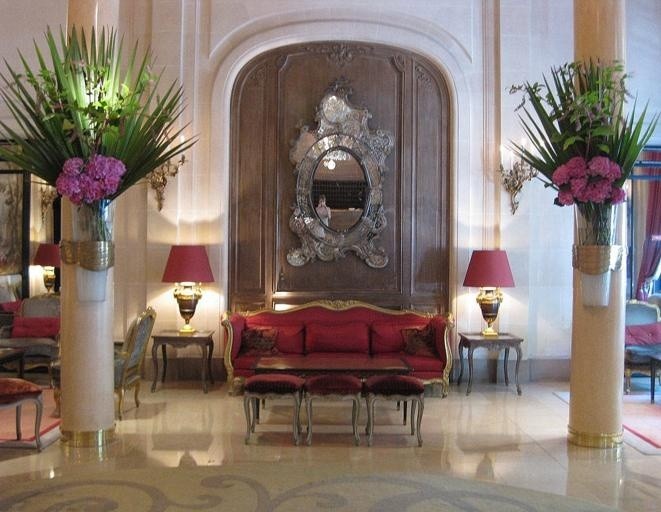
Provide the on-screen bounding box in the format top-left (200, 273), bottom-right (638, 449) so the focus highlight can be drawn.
top-left (1, 386), bottom-right (66, 445)
top-left (552, 385), bottom-right (661, 453)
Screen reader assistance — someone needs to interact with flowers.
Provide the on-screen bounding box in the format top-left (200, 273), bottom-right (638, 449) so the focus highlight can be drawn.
top-left (546, 149), bottom-right (632, 213)
top-left (55, 153), bottom-right (129, 205)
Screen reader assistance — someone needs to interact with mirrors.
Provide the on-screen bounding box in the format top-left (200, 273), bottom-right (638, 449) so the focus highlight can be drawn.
top-left (276, 78), bottom-right (397, 273)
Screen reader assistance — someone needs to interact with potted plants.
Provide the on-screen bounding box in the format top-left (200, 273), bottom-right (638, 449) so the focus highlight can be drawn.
top-left (0, 16), bottom-right (199, 305)
top-left (501, 53), bottom-right (660, 310)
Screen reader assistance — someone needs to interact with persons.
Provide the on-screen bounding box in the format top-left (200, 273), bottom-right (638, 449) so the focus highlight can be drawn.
top-left (314, 194), bottom-right (330, 229)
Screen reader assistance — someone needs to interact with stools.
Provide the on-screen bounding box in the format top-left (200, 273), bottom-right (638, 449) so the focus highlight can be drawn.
top-left (1, 376), bottom-right (47, 455)
top-left (240, 373), bottom-right (304, 446)
top-left (305, 373), bottom-right (363, 446)
top-left (363, 373), bottom-right (427, 448)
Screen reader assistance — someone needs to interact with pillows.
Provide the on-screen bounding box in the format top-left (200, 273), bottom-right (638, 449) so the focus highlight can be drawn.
top-left (398, 321), bottom-right (439, 360)
top-left (242, 325), bottom-right (280, 357)
top-left (11, 312), bottom-right (64, 339)
top-left (0, 299), bottom-right (21, 314)
top-left (623, 321), bottom-right (661, 347)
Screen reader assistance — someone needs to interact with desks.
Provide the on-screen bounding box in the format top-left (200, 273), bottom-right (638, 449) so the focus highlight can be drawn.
top-left (2, 344), bottom-right (29, 380)
top-left (146, 326), bottom-right (217, 394)
top-left (455, 329), bottom-right (527, 397)
top-left (253, 356), bottom-right (414, 427)
top-left (627, 340), bottom-right (661, 407)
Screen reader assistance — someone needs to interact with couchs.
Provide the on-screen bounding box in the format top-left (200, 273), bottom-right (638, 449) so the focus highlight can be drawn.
top-left (220, 297), bottom-right (457, 398)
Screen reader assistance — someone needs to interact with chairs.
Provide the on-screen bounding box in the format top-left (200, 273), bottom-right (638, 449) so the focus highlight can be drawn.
top-left (49, 303), bottom-right (160, 420)
top-left (618, 297), bottom-right (661, 395)
top-left (2, 292), bottom-right (66, 382)
top-left (0, 282), bottom-right (21, 330)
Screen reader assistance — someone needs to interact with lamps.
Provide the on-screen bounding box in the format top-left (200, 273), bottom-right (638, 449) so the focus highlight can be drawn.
top-left (322, 150), bottom-right (354, 170)
top-left (36, 179), bottom-right (58, 223)
top-left (462, 248), bottom-right (519, 337)
top-left (138, 136), bottom-right (189, 214)
top-left (160, 242), bottom-right (216, 333)
top-left (33, 241), bottom-right (66, 293)
top-left (497, 138), bottom-right (544, 215)
